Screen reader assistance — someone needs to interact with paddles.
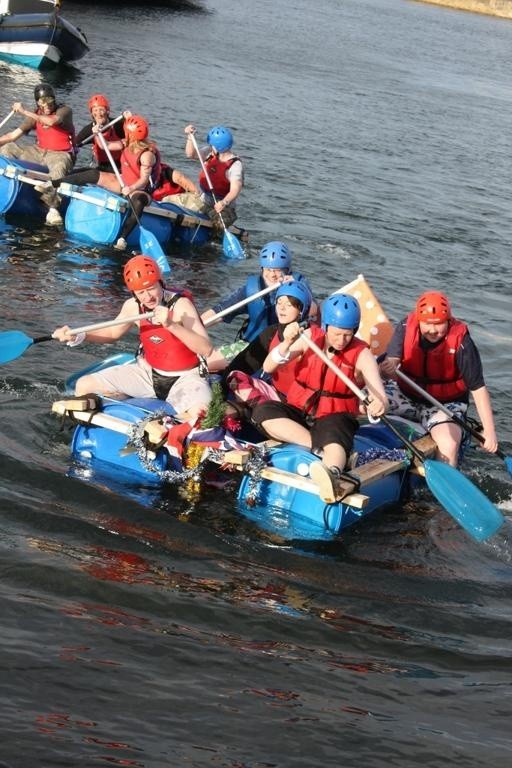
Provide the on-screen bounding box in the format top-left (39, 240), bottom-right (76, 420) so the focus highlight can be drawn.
top-left (57, 272), bottom-right (286, 399)
top-left (296, 321), bottom-right (506, 543)
top-left (190, 132), bottom-right (243, 261)
top-left (0, 311), bottom-right (155, 365)
top-left (97, 131), bottom-right (171, 273)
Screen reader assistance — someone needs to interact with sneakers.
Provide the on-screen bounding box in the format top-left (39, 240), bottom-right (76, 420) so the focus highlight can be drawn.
top-left (34, 181), bottom-right (54, 192)
top-left (308, 460), bottom-right (338, 504)
top-left (114, 238), bottom-right (126, 249)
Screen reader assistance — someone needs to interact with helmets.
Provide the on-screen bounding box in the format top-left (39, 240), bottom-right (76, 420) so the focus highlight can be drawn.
top-left (207, 125), bottom-right (233, 153)
top-left (274, 281), bottom-right (311, 321)
top-left (34, 83), bottom-right (55, 100)
top-left (124, 256), bottom-right (162, 292)
top-left (259, 241), bottom-right (291, 274)
top-left (124, 116), bottom-right (148, 140)
top-left (88, 95), bottom-right (110, 112)
top-left (321, 293), bottom-right (361, 333)
top-left (416, 293), bottom-right (452, 324)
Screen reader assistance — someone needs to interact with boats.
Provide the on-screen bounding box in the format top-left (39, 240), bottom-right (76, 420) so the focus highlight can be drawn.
top-left (1, 13), bottom-right (92, 72)
top-left (236, 416), bottom-right (477, 534)
top-left (1, 155), bottom-right (64, 222)
top-left (52, 383), bottom-right (248, 480)
top-left (56, 179), bottom-right (251, 253)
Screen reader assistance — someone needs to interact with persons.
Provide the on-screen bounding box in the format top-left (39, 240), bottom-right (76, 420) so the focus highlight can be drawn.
top-left (51, 243), bottom-right (500, 504)
top-left (1, 83), bottom-right (244, 244)
